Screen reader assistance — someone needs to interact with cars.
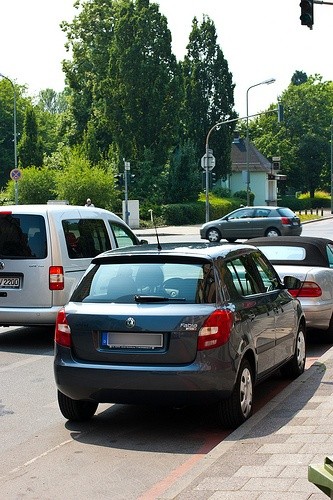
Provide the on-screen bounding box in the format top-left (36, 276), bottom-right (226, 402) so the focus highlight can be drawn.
top-left (242, 236), bottom-right (333, 330)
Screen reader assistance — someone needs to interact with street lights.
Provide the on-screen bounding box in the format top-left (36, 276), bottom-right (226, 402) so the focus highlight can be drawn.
top-left (245, 78), bottom-right (276, 206)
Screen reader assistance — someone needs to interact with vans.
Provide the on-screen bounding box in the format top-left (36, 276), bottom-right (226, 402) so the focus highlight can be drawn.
top-left (0, 205), bottom-right (149, 328)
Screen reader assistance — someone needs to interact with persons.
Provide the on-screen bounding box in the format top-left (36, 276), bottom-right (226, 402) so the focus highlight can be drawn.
top-left (85, 198), bottom-right (95, 207)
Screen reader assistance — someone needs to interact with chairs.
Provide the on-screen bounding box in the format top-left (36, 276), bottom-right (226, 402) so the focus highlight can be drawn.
top-left (107, 276), bottom-right (135, 297)
top-left (137, 266), bottom-right (163, 292)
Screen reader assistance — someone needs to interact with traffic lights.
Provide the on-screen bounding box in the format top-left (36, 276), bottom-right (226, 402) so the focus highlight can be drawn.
top-left (278, 105), bottom-right (284, 122)
top-left (299, 0), bottom-right (314, 30)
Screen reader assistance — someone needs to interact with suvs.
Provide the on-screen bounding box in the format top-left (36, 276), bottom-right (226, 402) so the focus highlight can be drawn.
top-left (51, 242), bottom-right (308, 423)
top-left (200, 206), bottom-right (302, 242)
